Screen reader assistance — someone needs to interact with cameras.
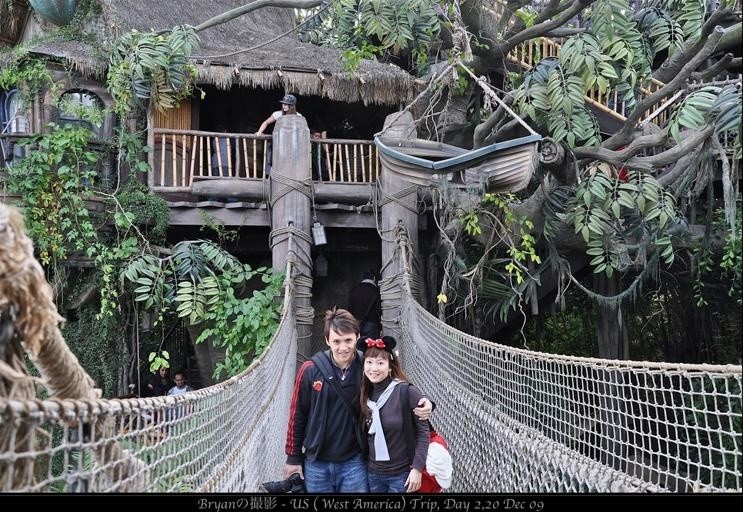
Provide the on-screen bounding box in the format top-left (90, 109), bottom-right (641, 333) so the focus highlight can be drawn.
top-left (261, 473), bottom-right (306, 495)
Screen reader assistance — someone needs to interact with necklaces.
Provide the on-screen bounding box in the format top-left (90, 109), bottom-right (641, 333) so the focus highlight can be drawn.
top-left (332, 360), bottom-right (352, 380)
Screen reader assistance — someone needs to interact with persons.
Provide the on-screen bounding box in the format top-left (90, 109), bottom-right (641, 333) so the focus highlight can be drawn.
top-left (360, 337), bottom-right (429, 492)
top-left (255, 94), bottom-right (304, 134)
top-left (346, 271), bottom-right (381, 350)
top-left (310, 130), bottom-right (321, 139)
top-left (148, 366), bottom-right (167, 394)
top-left (213, 126), bottom-right (232, 175)
top-left (167, 371), bottom-right (193, 396)
top-left (283, 305), bottom-right (437, 492)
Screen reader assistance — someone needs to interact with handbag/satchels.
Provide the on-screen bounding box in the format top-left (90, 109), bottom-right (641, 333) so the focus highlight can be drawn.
top-left (410, 431), bottom-right (453, 493)
top-left (354, 413), bottom-right (368, 453)
